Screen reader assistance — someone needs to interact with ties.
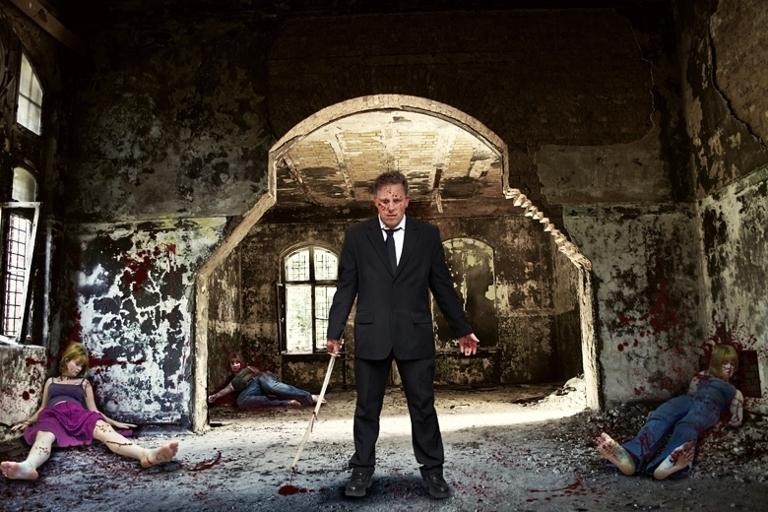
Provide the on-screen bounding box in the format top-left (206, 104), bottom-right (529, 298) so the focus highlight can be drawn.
top-left (380, 226), bottom-right (403, 271)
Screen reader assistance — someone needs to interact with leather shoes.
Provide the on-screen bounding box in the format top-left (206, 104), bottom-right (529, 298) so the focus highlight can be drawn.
top-left (345, 468), bottom-right (374, 497)
top-left (419, 464), bottom-right (451, 499)
